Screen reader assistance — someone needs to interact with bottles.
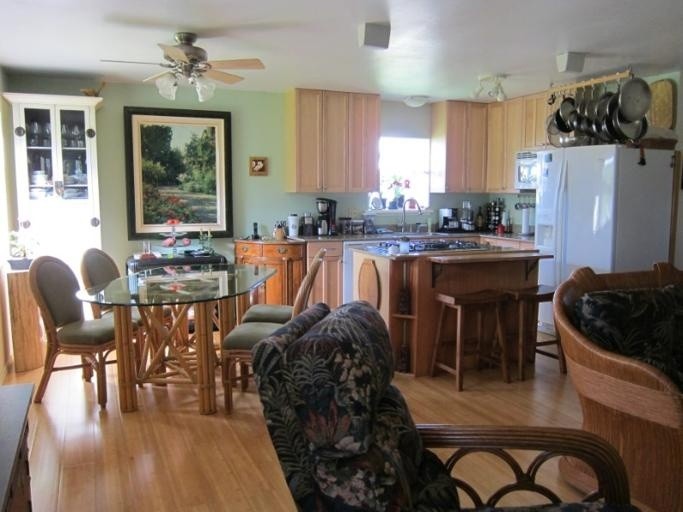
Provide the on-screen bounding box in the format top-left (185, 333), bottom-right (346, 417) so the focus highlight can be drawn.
top-left (476, 206), bottom-right (484, 230)
top-left (339, 212), bottom-right (377, 236)
top-left (497, 224), bottom-right (505, 234)
top-left (273, 212), bottom-right (314, 241)
top-left (26, 122), bottom-right (49, 147)
top-left (61, 123), bottom-right (84, 147)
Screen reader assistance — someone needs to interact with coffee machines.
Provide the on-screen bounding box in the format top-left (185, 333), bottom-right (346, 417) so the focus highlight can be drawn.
top-left (438, 207), bottom-right (461, 233)
top-left (316, 197), bottom-right (337, 235)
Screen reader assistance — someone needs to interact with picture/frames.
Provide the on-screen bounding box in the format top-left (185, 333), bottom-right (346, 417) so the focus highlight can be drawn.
top-left (123, 106), bottom-right (233, 241)
top-left (248, 156), bottom-right (267, 176)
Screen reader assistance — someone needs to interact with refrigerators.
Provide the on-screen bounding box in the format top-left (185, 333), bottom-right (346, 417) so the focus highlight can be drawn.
top-left (535, 145), bottom-right (675, 337)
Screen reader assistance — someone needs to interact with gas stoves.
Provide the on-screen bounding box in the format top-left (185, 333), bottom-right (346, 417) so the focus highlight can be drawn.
top-left (374, 239), bottom-right (488, 253)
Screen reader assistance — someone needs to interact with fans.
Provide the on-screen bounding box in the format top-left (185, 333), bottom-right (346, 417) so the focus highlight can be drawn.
top-left (101, 42), bottom-right (266, 88)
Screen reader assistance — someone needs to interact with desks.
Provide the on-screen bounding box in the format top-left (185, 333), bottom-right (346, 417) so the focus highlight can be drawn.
top-left (350, 243), bottom-right (554, 378)
top-left (121, 248), bottom-right (227, 274)
top-left (1, 383), bottom-right (35, 511)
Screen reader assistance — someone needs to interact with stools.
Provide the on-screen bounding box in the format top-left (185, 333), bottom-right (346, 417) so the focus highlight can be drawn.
top-left (505, 284), bottom-right (567, 379)
top-left (431, 288), bottom-right (508, 392)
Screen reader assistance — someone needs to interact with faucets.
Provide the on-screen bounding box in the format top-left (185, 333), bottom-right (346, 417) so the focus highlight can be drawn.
top-left (402, 198), bottom-right (423, 232)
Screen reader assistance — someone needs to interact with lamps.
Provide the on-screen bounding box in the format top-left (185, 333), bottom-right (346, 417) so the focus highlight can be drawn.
top-left (156, 32), bottom-right (216, 103)
top-left (472, 73), bottom-right (508, 103)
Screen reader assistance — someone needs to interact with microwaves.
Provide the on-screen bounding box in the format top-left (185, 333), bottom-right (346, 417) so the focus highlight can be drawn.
top-left (514, 150), bottom-right (536, 190)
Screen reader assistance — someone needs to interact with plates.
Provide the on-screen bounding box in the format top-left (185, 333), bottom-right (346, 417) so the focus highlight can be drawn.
top-left (371, 197), bottom-right (381, 208)
top-left (64, 176), bottom-right (84, 199)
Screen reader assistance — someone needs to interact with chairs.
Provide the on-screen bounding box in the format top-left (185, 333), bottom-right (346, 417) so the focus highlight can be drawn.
top-left (251, 300), bottom-right (642, 511)
top-left (552, 261), bottom-right (683, 512)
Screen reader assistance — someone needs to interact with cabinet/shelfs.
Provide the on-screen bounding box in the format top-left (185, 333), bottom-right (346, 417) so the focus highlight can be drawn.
top-left (4, 90), bottom-right (102, 288)
top-left (298, 91), bottom-right (380, 194)
top-left (308, 242), bottom-right (344, 310)
top-left (430, 97), bottom-right (543, 196)
top-left (237, 242), bottom-right (305, 305)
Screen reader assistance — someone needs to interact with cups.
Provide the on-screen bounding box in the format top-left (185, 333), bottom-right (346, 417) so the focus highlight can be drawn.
top-left (142, 241), bottom-right (151, 254)
top-left (400, 237), bottom-right (410, 254)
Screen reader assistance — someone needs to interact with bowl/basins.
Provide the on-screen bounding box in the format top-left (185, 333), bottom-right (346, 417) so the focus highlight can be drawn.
top-left (30, 170), bottom-right (49, 199)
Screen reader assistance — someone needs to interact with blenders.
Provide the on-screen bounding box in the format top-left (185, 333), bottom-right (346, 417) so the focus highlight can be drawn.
top-left (460, 200), bottom-right (476, 231)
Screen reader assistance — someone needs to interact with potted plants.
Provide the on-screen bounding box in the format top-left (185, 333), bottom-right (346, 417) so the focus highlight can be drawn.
top-left (7, 233), bottom-right (33, 270)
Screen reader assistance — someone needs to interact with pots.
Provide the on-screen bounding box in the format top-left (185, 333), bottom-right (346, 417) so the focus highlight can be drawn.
top-left (546, 75), bottom-right (652, 147)
